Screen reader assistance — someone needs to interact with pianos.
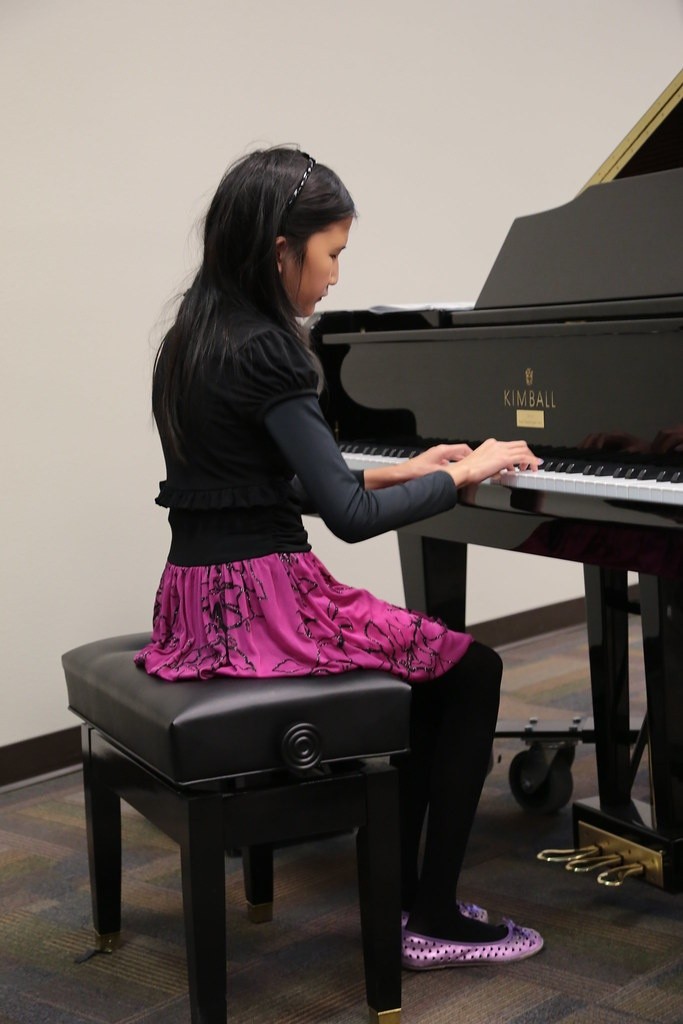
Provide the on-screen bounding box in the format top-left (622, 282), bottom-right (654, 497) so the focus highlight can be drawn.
top-left (299, 63), bottom-right (683, 894)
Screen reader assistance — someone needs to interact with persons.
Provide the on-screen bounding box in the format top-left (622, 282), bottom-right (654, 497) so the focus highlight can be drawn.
top-left (133, 145), bottom-right (547, 970)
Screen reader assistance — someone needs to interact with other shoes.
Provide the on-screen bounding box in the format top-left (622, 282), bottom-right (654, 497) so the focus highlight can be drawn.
top-left (402, 899), bottom-right (488, 929)
top-left (401, 917), bottom-right (544, 969)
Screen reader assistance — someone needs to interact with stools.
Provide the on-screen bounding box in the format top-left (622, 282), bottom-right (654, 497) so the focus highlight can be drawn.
top-left (63, 632), bottom-right (415, 1024)
